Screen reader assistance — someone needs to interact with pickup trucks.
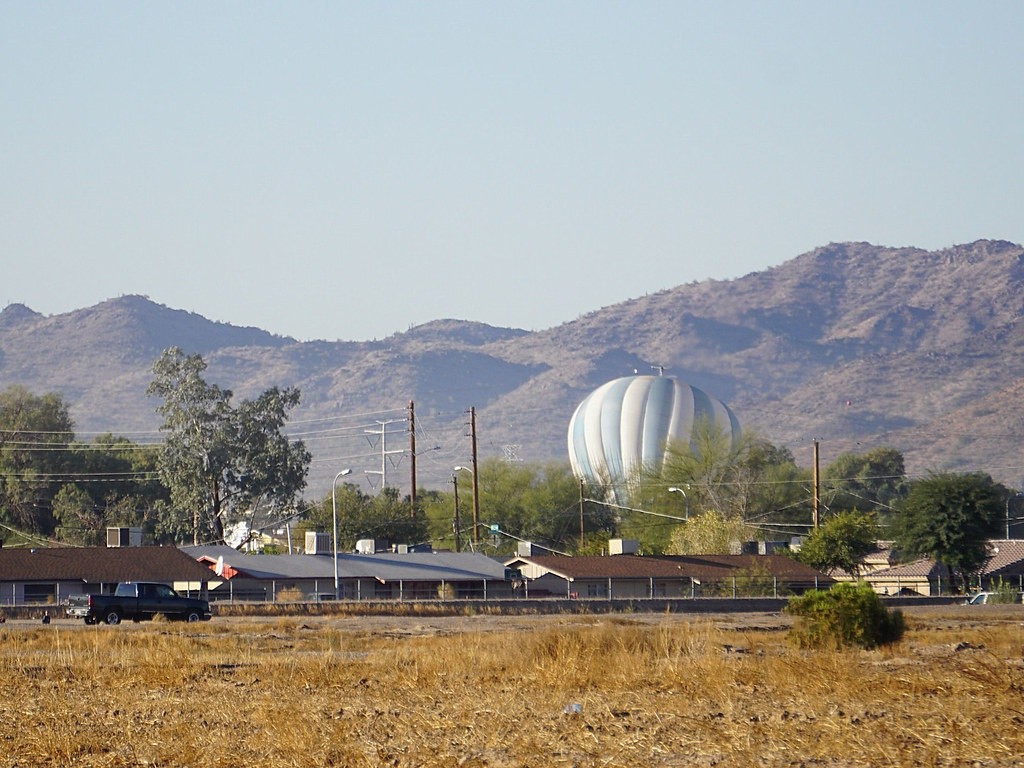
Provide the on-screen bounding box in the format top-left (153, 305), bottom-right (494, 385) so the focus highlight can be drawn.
top-left (68, 581), bottom-right (213, 625)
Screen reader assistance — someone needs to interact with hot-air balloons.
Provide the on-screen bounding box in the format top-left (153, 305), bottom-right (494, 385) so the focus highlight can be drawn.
top-left (568, 374), bottom-right (742, 523)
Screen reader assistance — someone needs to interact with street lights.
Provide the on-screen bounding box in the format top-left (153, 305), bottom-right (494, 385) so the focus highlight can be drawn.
top-left (668, 486), bottom-right (688, 522)
top-left (333, 469), bottom-right (352, 601)
top-left (455, 465), bottom-right (472, 474)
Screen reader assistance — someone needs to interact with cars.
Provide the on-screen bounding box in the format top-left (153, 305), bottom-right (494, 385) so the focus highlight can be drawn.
top-left (962, 592), bottom-right (1000, 606)
